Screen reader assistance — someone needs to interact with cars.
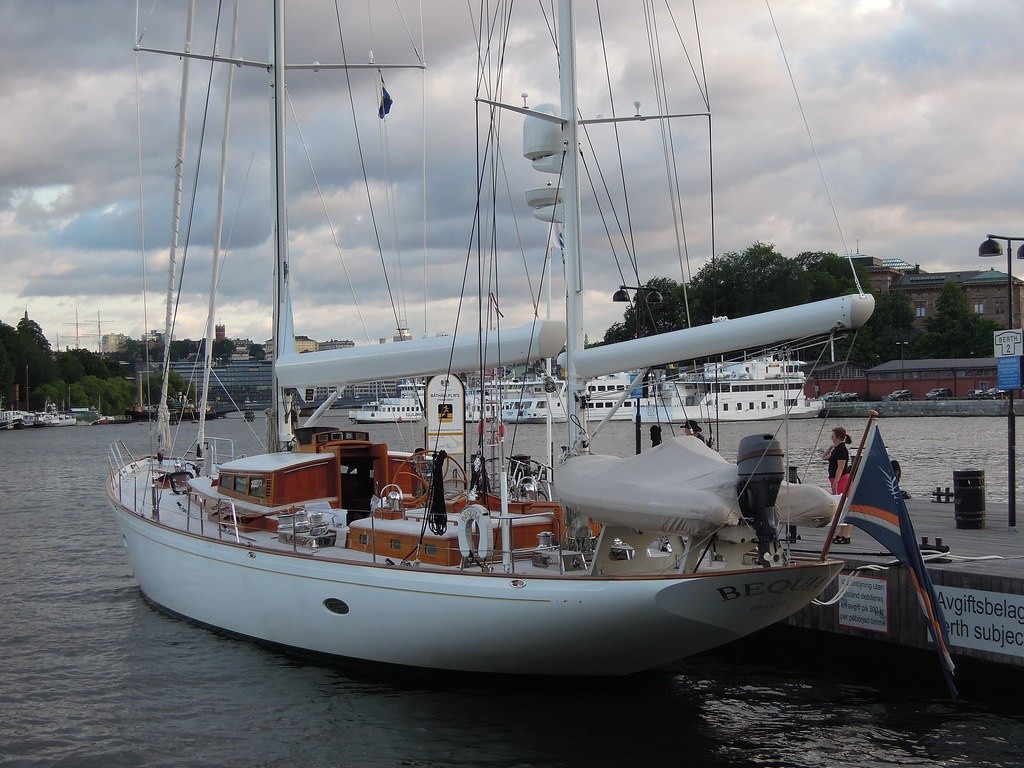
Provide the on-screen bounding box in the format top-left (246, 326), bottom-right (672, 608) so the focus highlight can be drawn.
top-left (888, 390), bottom-right (912, 401)
top-left (838, 393), bottom-right (861, 402)
top-left (967, 389), bottom-right (986, 400)
top-left (982, 388), bottom-right (1010, 400)
top-left (821, 390), bottom-right (842, 402)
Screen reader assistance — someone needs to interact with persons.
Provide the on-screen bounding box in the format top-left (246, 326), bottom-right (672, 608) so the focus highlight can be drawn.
top-left (680, 420), bottom-right (705, 443)
top-left (821, 427), bottom-right (852, 544)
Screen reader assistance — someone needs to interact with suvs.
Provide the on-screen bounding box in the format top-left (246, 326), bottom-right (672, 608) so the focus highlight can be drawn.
top-left (926, 388), bottom-right (953, 400)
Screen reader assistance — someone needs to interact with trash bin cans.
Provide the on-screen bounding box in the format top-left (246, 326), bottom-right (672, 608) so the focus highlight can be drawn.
top-left (953, 465), bottom-right (986, 530)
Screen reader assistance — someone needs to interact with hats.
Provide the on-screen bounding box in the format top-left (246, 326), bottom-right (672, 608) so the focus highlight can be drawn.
top-left (680, 420), bottom-right (702, 432)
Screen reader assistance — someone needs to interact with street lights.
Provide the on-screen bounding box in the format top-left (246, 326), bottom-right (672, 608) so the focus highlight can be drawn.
top-left (68, 385), bottom-right (71, 410)
top-left (613, 286), bottom-right (664, 455)
top-left (979, 234), bottom-right (1023, 527)
top-left (896, 341), bottom-right (909, 391)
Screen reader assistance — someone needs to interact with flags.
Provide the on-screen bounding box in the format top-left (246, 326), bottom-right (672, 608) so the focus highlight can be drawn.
top-left (838, 418), bottom-right (957, 675)
top-left (378, 71), bottom-right (393, 119)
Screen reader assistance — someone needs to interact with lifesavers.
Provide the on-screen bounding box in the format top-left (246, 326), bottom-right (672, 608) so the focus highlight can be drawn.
top-left (477, 415), bottom-right (507, 445)
top-left (731, 371), bottom-right (736, 378)
top-left (456, 503), bottom-right (495, 560)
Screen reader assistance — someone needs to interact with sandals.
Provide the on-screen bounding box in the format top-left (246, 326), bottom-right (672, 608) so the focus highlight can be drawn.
top-left (833, 536), bottom-right (851, 545)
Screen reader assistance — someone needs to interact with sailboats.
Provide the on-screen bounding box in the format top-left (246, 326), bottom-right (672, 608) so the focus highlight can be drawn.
top-left (106, 1), bottom-right (876, 681)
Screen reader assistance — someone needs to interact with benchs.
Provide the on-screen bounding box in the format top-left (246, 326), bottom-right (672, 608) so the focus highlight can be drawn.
top-left (186, 476), bottom-right (338, 534)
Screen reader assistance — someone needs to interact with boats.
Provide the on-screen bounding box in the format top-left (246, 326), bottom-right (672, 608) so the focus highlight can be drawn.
top-left (0, 410), bottom-right (77, 430)
top-left (191, 419), bottom-right (199, 423)
top-left (348, 375), bottom-right (632, 425)
top-left (244, 410), bottom-right (255, 422)
top-left (631, 361), bottom-right (826, 424)
